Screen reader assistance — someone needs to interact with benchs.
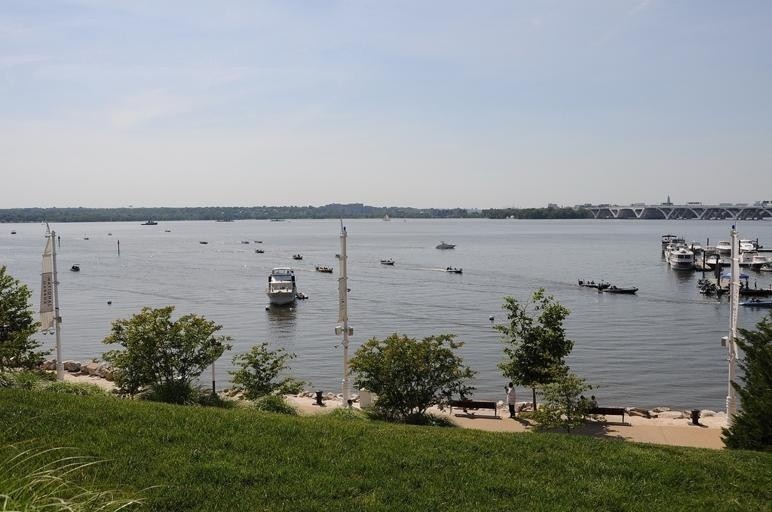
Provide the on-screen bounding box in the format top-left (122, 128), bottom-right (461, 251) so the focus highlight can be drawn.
top-left (448, 400), bottom-right (497, 419)
top-left (575, 406), bottom-right (625, 425)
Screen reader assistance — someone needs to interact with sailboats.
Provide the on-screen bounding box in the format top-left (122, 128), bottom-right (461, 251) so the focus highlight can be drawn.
top-left (383, 212), bottom-right (392, 221)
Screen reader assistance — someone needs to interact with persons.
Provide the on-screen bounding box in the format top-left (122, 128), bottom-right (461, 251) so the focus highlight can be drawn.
top-left (588, 394), bottom-right (598, 407)
top-left (577, 394), bottom-right (588, 405)
top-left (505, 381), bottom-right (518, 417)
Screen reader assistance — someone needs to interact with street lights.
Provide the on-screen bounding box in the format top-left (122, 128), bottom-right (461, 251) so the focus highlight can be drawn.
top-left (333, 324), bottom-right (354, 408)
top-left (720, 335), bottom-right (746, 429)
top-left (42, 317), bottom-right (64, 381)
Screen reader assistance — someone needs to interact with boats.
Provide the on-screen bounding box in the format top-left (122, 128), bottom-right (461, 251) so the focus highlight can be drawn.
top-left (297, 293), bottom-right (308, 300)
top-left (380, 259), bottom-right (395, 266)
top-left (435, 240), bottom-right (454, 249)
top-left (445, 266), bottom-right (463, 273)
top-left (267, 266), bottom-right (297, 305)
top-left (660, 233), bottom-right (772, 309)
top-left (199, 239), bottom-right (334, 273)
top-left (577, 278), bottom-right (612, 290)
top-left (600, 286), bottom-right (639, 293)
top-left (141, 218), bottom-right (157, 226)
top-left (70, 264), bottom-right (80, 272)
